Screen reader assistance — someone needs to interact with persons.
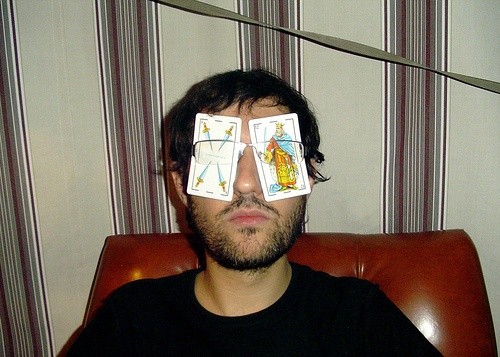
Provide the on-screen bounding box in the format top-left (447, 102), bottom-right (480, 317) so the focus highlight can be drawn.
top-left (54, 68), bottom-right (445, 357)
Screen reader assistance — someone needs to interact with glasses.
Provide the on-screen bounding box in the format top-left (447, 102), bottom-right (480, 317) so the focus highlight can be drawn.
top-left (190, 140), bottom-right (310, 165)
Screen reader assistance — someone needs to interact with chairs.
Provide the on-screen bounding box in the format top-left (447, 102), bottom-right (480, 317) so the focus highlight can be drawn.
top-left (58, 229), bottom-right (498, 357)
